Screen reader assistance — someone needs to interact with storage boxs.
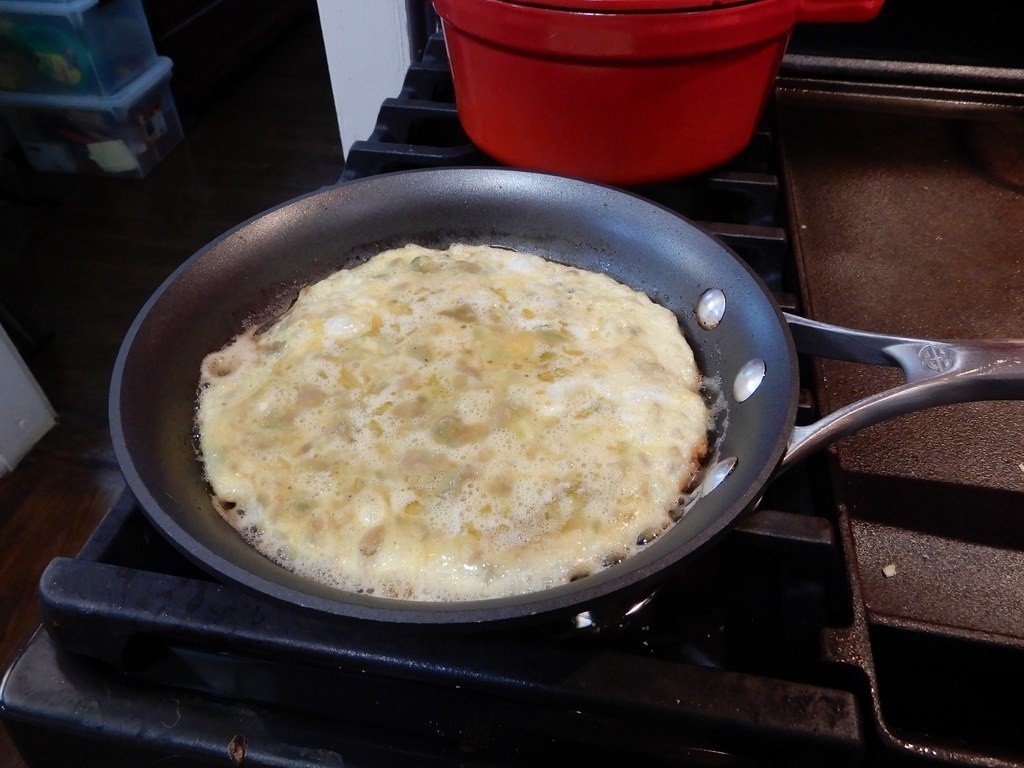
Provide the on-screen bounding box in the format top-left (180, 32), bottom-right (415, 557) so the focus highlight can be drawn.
top-left (0, 0), bottom-right (184, 180)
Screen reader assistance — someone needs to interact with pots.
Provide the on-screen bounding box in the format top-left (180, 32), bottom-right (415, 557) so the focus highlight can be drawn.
top-left (433, 0), bottom-right (881, 186)
top-left (106, 167), bottom-right (1024, 622)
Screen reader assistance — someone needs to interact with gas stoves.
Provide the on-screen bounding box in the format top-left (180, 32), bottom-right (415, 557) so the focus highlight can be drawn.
top-left (1, 68), bottom-right (921, 766)
